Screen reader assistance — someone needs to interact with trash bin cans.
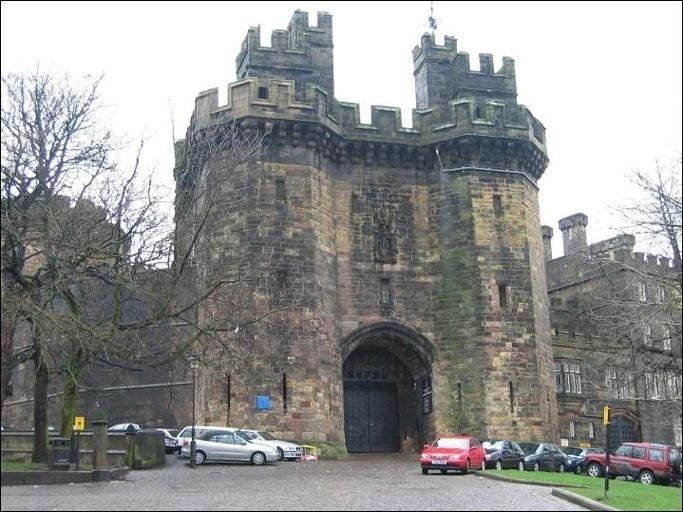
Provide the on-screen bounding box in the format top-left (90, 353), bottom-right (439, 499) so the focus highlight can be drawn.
top-left (47, 437), bottom-right (71, 471)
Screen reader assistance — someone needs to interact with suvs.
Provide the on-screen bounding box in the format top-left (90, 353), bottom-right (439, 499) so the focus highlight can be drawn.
top-left (580, 437), bottom-right (681, 488)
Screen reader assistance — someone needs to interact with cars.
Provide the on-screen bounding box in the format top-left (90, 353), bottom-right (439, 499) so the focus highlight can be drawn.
top-left (479, 437), bottom-right (605, 477)
top-left (104, 420), bottom-right (303, 469)
top-left (416, 433), bottom-right (487, 476)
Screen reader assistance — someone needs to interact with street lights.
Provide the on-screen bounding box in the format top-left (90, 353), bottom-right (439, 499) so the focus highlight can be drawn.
top-left (188, 355), bottom-right (199, 470)
